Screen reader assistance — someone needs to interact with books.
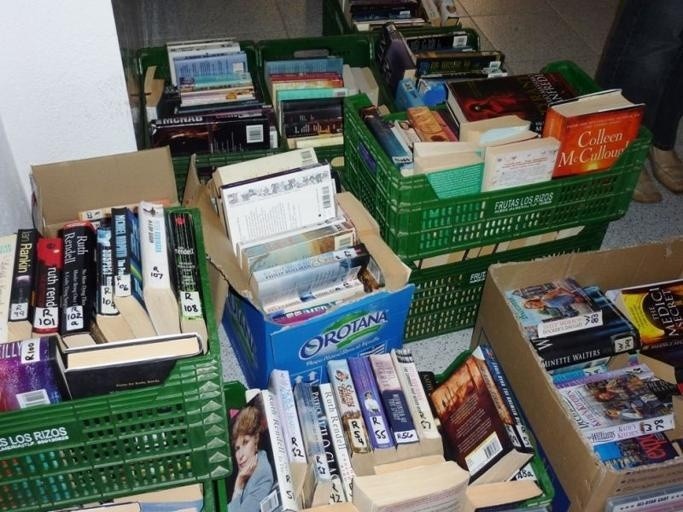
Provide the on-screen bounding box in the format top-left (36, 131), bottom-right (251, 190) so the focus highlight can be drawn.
top-left (1, 1), bottom-right (682, 512)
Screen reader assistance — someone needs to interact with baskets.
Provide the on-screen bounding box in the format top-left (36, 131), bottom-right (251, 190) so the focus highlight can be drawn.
top-left (214, 348), bottom-right (556, 512)
top-left (322, 1), bottom-right (461, 35)
top-left (254, 32), bottom-right (396, 163)
top-left (1, 206), bottom-right (234, 512)
top-left (403, 220), bottom-right (610, 343)
top-left (341, 60), bottom-right (655, 259)
top-left (134, 40), bottom-right (291, 205)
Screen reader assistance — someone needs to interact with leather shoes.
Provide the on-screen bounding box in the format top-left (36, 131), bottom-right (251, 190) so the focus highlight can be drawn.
top-left (648, 143), bottom-right (682, 194)
top-left (632, 164), bottom-right (661, 203)
